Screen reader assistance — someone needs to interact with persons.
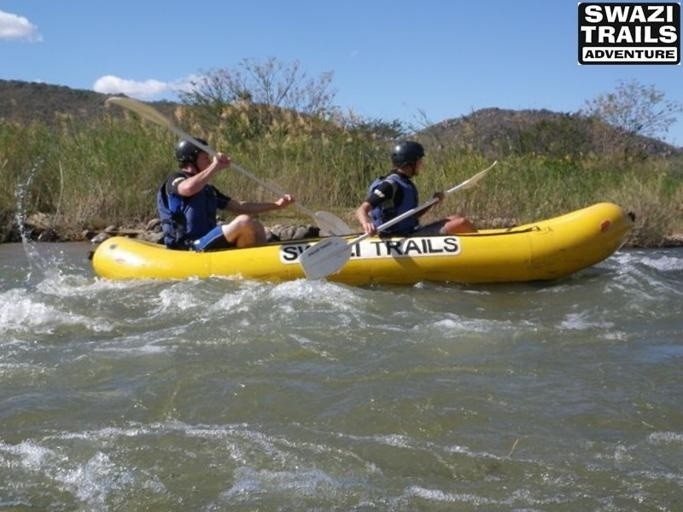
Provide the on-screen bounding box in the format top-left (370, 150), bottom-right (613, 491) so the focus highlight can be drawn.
top-left (355, 141), bottom-right (479, 239)
top-left (156, 137), bottom-right (295, 252)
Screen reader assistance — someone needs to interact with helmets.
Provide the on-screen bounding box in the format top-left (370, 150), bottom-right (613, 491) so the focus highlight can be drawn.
top-left (176, 137), bottom-right (208, 161)
top-left (392, 142), bottom-right (424, 168)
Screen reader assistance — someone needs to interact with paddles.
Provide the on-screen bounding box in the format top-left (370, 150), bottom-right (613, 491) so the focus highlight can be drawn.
top-left (297, 160), bottom-right (500, 281)
top-left (108, 96), bottom-right (350, 235)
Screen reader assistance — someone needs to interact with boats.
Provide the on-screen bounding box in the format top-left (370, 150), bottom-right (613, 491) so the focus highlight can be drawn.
top-left (92, 201), bottom-right (633, 286)
